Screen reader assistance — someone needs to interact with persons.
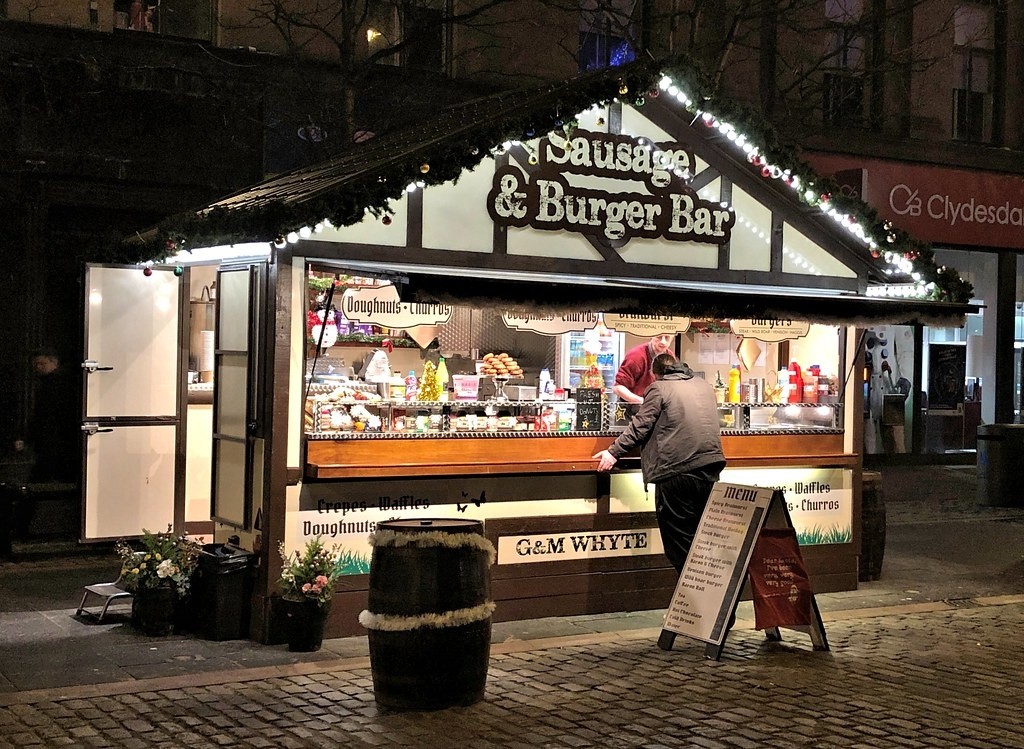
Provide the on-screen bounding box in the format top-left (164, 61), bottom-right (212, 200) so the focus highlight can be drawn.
top-left (864, 326), bottom-right (914, 454)
top-left (591, 353), bottom-right (727, 577)
top-left (612, 334), bottom-right (677, 426)
top-left (10, 347), bottom-right (79, 536)
top-left (939, 378), bottom-right (961, 410)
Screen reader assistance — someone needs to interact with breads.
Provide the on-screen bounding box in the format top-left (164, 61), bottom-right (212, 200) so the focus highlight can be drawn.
top-left (480, 352), bottom-right (523, 375)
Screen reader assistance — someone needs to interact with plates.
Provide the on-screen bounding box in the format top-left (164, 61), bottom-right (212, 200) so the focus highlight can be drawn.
top-left (480, 373), bottom-right (525, 381)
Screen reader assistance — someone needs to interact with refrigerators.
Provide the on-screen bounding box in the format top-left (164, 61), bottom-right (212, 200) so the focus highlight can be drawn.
top-left (554, 316), bottom-right (625, 389)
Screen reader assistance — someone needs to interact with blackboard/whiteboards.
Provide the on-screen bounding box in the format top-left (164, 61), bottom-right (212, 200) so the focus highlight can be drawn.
top-left (576, 387), bottom-right (601, 431)
top-left (928, 344), bottom-right (967, 415)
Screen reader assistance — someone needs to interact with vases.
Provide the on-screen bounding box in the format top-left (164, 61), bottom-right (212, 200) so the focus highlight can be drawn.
top-left (282, 597), bottom-right (329, 652)
top-left (131, 585), bottom-right (182, 636)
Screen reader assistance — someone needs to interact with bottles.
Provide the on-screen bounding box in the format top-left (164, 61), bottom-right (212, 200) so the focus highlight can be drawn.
top-left (538, 368), bottom-right (565, 401)
top-left (210, 280), bottom-right (217, 300)
top-left (393, 404), bottom-right (444, 433)
top-left (434, 357), bottom-right (450, 402)
top-left (776, 357), bottom-right (830, 404)
top-left (320, 410), bottom-right (330, 430)
top-left (715, 364), bottom-right (766, 403)
top-left (569, 321), bottom-right (616, 367)
top-left (388, 370), bottom-right (406, 399)
top-left (404, 370), bottom-right (417, 402)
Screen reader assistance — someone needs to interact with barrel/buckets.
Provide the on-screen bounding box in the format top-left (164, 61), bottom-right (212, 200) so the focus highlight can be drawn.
top-left (452, 373), bottom-right (480, 400)
top-left (367, 517), bottom-right (493, 713)
top-left (856, 467), bottom-right (887, 583)
top-left (975, 422), bottom-right (1024, 509)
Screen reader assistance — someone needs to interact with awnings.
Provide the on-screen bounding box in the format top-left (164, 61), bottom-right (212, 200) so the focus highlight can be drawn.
top-left (314, 259), bottom-right (987, 334)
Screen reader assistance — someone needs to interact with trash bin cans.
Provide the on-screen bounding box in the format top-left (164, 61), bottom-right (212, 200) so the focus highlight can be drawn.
top-left (190, 542), bottom-right (256, 641)
top-left (977, 423), bottom-right (1024, 506)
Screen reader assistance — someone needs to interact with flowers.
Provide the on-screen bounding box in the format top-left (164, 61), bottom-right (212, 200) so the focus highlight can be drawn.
top-left (114, 526), bottom-right (201, 597)
top-left (277, 533), bottom-right (347, 602)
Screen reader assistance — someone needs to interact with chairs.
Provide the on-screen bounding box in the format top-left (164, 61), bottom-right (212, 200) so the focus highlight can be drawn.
top-left (75, 551), bottom-right (150, 624)
